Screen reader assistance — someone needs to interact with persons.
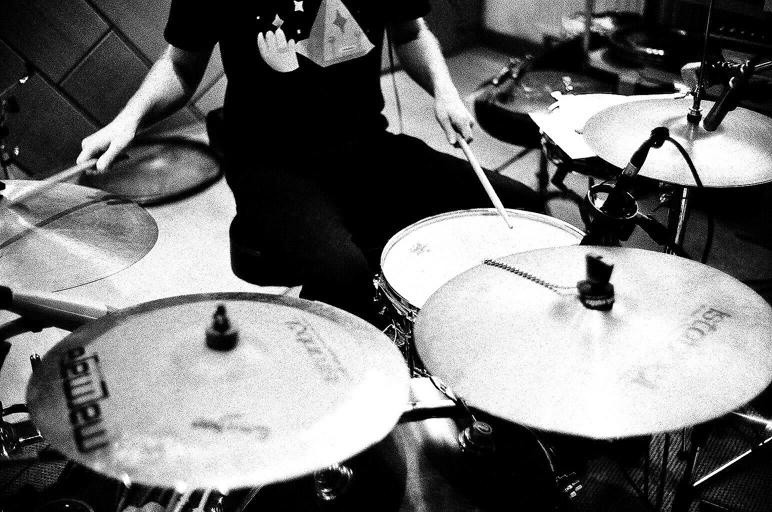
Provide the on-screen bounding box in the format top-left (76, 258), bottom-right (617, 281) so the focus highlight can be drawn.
top-left (74, 2), bottom-right (551, 351)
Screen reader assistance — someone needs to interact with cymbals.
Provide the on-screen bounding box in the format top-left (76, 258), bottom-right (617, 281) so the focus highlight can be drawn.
top-left (412, 243), bottom-right (772, 439)
top-left (582, 99), bottom-right (771, 188)
top-left (26, 292), bottom-right (412, 492)
top-left (0, 178), bottom-right (158, 296)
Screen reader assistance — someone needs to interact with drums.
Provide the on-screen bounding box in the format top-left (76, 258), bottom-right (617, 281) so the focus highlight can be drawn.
top-left (308, 374), bottom-right (583, 511)
top-left (371, 205), bottom-right (586, 372)
